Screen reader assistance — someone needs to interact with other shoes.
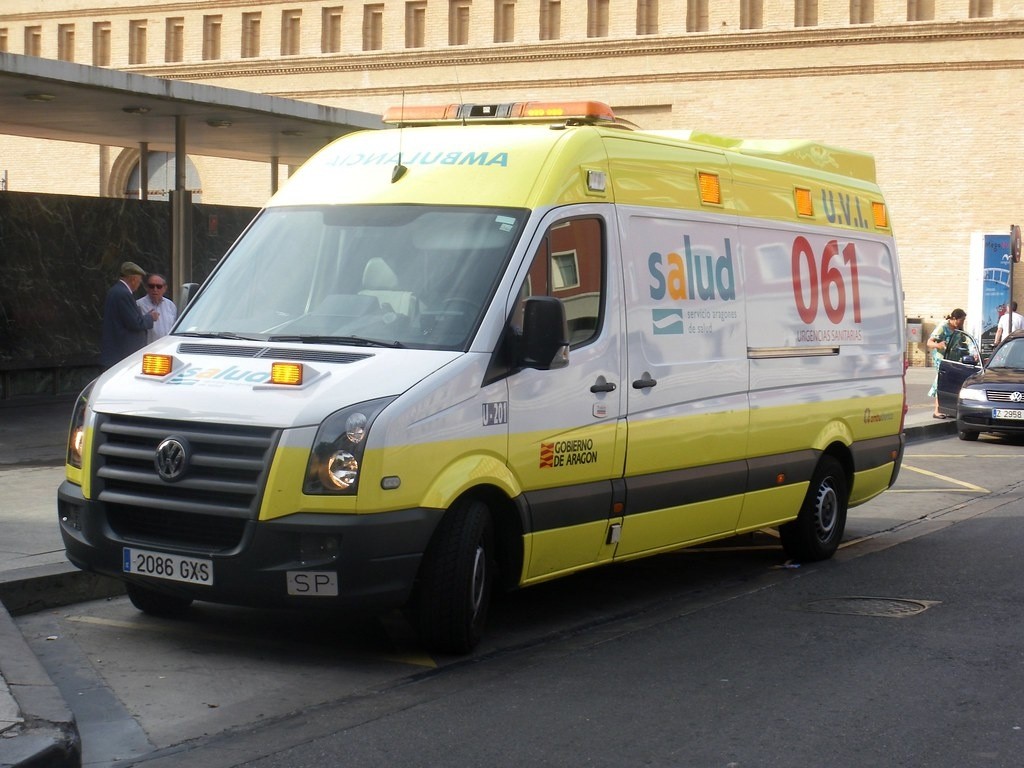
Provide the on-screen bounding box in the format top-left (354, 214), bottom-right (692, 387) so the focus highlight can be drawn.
top-left (933, 413), bottom-right (946, 419)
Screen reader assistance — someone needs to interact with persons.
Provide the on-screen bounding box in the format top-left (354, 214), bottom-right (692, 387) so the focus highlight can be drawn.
top-left (927, 308), bottom-right (970, 419)
top-left (136, 274), bottom-right (178, 345)
top-left (101, 262), bottom-right (160, 369)
top-left (995, 301), bottom-right (1024, 346)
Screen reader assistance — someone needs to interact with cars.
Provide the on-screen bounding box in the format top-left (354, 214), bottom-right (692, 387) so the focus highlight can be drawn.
top-left (935, 328), bottom-right (1024, 444)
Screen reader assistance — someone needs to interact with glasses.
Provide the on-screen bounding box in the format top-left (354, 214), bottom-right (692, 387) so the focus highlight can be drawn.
top-left (148, 284), bottom-right (167, 289)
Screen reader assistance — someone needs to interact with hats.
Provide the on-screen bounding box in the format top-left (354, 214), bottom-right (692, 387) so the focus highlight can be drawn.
top-left (120, 262), bottom-right (146, 277)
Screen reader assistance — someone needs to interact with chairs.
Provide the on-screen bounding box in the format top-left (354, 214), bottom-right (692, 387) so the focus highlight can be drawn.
top-left (361, 257), bottom-right (423, 318)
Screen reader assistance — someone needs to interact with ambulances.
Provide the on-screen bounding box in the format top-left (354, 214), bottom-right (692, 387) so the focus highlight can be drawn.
top-left (53, 99), bottom-right (914, 658)
